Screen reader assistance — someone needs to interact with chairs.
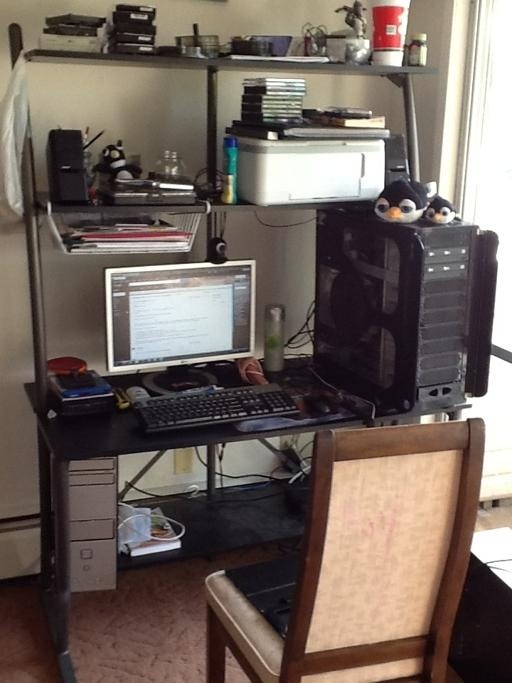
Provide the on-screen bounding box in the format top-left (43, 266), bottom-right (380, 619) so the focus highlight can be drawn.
top-left (207, 417), bottom-right (486, 683)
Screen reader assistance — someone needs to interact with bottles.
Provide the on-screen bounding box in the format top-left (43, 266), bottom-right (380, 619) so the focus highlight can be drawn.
top-left (157, 148), bottom-right (185, 181)
top-left (262, 305), bottom-right (285, 373)
top-left (408, 32), bottom-right (428, 65)
top-left (220, 136), bottom-right (240, 204)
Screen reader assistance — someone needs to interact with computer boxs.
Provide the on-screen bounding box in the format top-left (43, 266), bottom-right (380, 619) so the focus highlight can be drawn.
top-left (68, 456), bottom-right (119, 593)
top-left (311, 199), bottom-right (479, 414)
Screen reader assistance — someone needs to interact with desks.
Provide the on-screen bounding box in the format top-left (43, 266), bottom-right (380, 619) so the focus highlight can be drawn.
top-left (23, 358), bottom-right (473, 683)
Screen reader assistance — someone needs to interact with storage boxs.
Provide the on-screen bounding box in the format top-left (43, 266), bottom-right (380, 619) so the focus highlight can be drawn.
top-left (235, 136), bottom-right (385, 206)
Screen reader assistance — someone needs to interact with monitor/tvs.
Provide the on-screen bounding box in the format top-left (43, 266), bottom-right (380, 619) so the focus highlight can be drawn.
top-left (103, 258), bottom-right (257, 396)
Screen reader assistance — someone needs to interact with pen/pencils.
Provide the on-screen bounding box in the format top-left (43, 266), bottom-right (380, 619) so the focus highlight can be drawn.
top-left (82, 126), bottom-right (90, 143)
top-left (82, 130), bottom-right (105, 151)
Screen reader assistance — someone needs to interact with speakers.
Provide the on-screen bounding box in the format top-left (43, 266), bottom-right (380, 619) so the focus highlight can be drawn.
top-left (45, 129), bottom-right (86, 205)
top-left (384, 134), bottom-right (409, 188)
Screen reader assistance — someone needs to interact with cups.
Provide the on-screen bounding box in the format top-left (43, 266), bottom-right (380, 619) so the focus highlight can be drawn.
top-left (372, 1), bottom-right (411, 68)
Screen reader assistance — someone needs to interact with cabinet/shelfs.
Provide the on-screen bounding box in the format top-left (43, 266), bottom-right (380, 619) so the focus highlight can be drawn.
top-left (8, 25), bottom-right (438, 212)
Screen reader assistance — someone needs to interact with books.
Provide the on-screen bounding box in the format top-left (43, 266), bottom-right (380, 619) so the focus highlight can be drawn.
top-left (241, 77), bottom-right (306, 123)
top-left (65, 213), bottom-right (198, 252)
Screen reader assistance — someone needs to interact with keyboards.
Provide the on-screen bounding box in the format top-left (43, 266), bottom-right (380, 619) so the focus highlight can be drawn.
top-left (130, 380), bottom-right (303, 435)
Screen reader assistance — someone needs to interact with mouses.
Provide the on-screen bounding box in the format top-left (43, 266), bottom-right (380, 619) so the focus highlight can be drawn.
top-left (303, 395), bottom-right (332, 414)
